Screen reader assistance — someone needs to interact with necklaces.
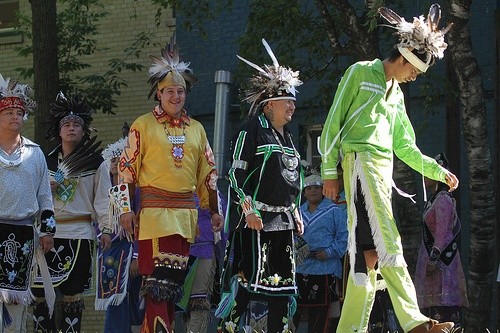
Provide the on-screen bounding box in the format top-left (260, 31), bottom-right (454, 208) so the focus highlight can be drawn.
top-left (163, 121), bottom-right (185, 168)
top-left (0, 137), bottom-right (25, 166)
top-left (272, 128), bottom-right (298, 181)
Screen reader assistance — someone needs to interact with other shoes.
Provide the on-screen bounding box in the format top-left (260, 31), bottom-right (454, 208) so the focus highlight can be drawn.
top-left (408, 319), bottom-right (455, 333)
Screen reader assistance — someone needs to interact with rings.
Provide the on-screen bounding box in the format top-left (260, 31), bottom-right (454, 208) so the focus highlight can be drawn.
top-left (451, 183), bottom-right (454, 187)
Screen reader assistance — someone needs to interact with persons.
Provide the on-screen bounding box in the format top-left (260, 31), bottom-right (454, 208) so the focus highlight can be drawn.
top-left (27, 91), bottom-right (111, 333)
top-left (93, 43), bottom-right (224, 333)
top-left (215, 38), bottom-right (304, 333)
top-left (0, 72), bottom-right (56, 333)
top-left (294, 3), bottom-right (469, 333)
